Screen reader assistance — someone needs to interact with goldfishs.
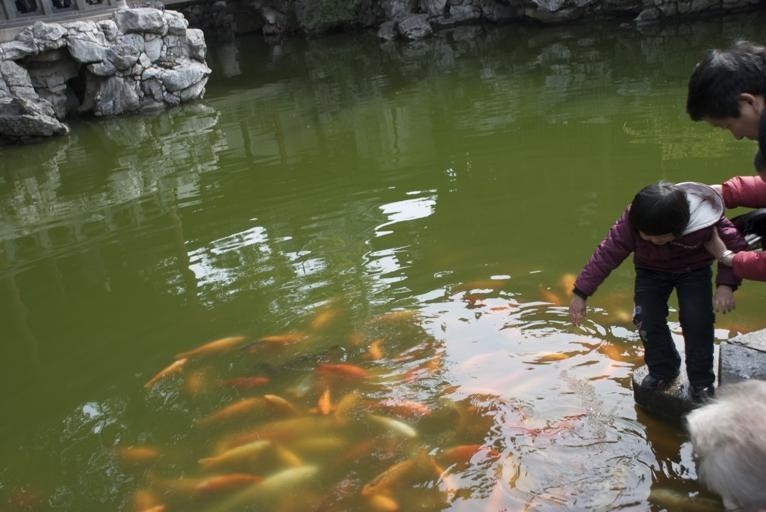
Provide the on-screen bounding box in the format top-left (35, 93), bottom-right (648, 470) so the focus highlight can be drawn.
top-left (110, 271), bottom-right (752, 512)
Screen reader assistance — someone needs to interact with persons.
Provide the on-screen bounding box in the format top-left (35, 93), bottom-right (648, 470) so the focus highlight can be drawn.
top-left (569, 181), bottom-right (750, 405)
top-left (703, 152), bottom-right (765, 281)
top-left (686, 40), bottom-right (766, 144)
top-left (685, 380), bottom-right (766, 511)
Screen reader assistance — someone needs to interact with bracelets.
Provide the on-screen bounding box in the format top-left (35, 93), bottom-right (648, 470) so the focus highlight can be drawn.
top-left (717, 249), bottom-right (734, 262)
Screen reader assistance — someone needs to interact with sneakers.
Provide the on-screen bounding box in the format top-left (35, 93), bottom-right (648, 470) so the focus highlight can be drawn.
top-left (688, 385), bottom-right (714, 403)
top-left (641, 369), bottom-right (680, 389)
